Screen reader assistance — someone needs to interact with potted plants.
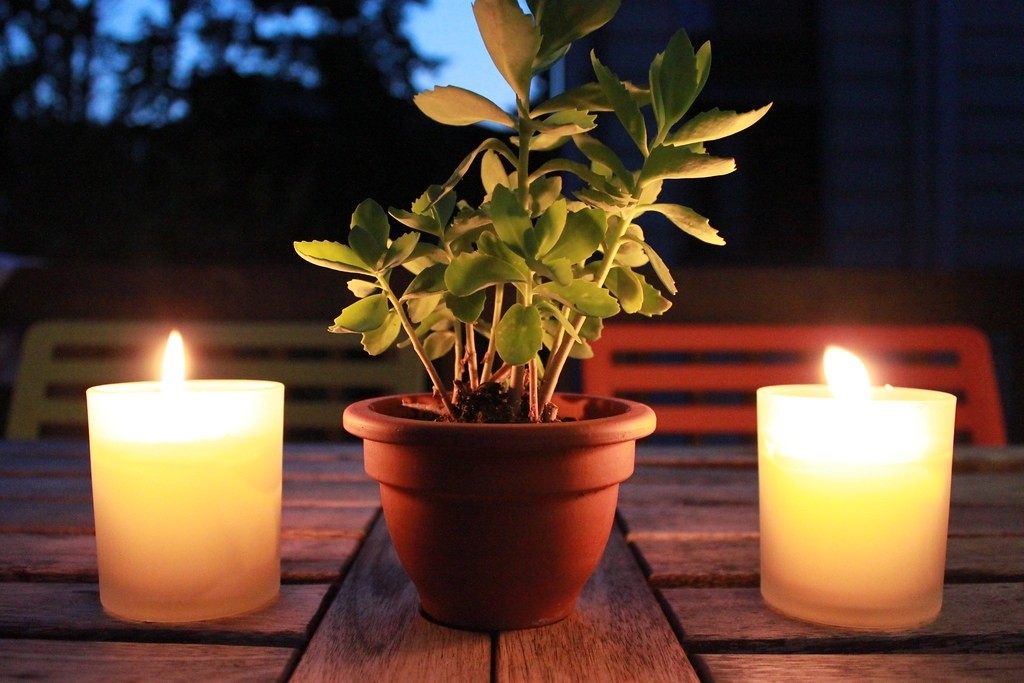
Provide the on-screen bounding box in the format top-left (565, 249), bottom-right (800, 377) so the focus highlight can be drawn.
top-left (291, 0), bottom-right (773, 633)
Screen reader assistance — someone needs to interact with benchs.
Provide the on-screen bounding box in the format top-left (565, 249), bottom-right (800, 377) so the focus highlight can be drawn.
top-left (7, 321), bottom-right (423, 444)
top-left (585, 324), bottom-right (1006, 448)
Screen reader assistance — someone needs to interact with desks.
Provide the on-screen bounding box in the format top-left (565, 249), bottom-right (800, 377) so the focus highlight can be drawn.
top-left (494, 446), bottom-right (1024, 683)
top-left (0, 438), bottom-right (495, 683)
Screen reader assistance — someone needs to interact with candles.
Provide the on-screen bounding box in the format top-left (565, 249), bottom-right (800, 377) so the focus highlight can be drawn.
top-left (85, 328), bottom-right (284, 628)
top-left (757, 344), bottom-right (957, 633)
